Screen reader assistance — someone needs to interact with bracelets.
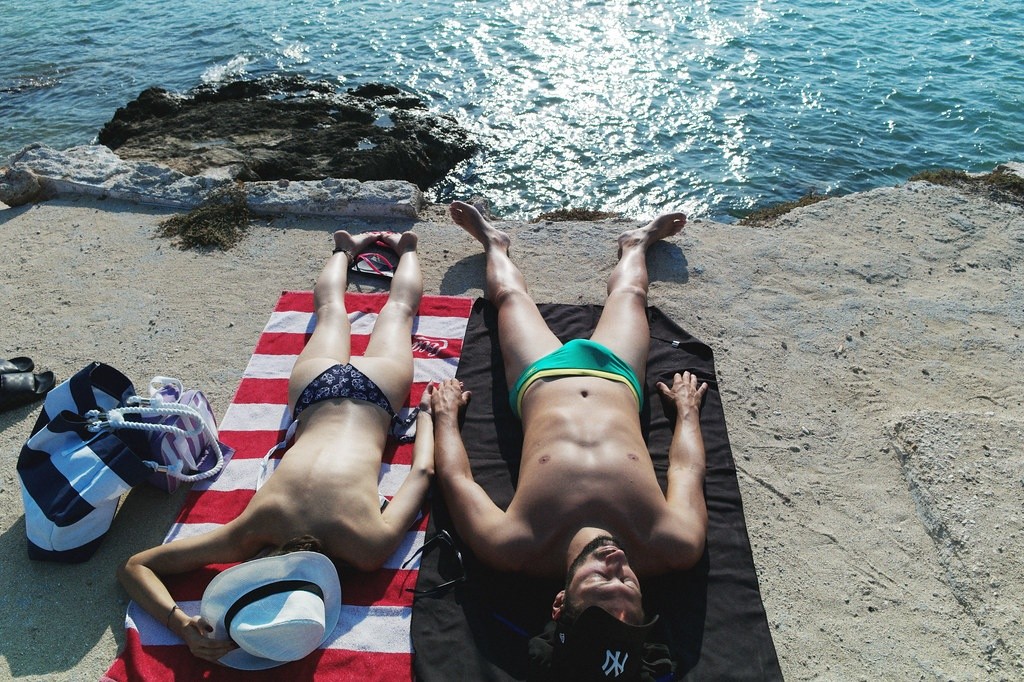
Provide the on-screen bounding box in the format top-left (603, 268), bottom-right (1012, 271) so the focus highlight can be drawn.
top-left (166, 605), bottom-right (181, 631)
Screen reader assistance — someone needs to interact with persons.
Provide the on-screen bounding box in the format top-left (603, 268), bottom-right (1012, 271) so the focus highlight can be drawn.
top-left (430, 200), bottom-right (710, 682)
top-left (118, 230), bottom-right (435, 671)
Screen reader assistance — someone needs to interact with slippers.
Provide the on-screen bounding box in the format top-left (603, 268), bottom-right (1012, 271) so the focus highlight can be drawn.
top-left (366, 228), bottom-right (400, 250)
top-left (0, 356), bottom-right (56, 409)
top-left (349, 252), bottom-right (398, 280)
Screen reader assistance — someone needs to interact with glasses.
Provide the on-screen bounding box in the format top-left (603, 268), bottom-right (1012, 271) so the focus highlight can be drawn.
top-left (400, 526), bottom-right (469, 595)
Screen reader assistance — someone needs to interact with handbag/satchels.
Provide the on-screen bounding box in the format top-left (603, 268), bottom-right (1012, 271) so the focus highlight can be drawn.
top-left (139, 373), bottom-right (220, 493)
top-left (18, 360), bottom-right (225, 567)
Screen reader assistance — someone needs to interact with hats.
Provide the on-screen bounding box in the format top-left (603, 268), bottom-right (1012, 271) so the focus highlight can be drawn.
top-left (553, 605), bottom-right (663, 682)
top-left (201, 552), bottom-right (343, 672)
